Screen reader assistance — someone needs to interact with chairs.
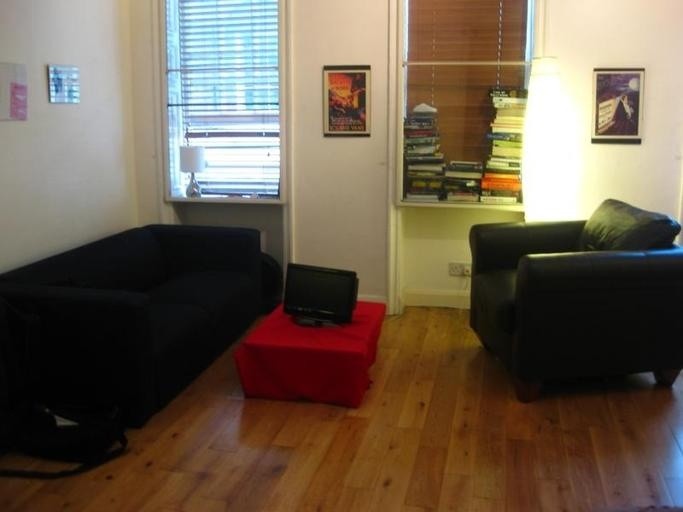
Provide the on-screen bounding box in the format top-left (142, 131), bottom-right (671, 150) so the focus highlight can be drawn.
top-left (467, 199), bottom-right (683, 404)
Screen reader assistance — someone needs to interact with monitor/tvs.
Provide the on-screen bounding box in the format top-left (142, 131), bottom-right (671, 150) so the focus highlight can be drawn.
top-left (282, 264), bottom-right (359, 328)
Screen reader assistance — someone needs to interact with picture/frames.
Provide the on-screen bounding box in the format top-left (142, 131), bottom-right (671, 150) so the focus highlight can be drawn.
top-left (589, 67), bottom-right (646, 145)
top-left (321, 65), bottom-right (372, 138)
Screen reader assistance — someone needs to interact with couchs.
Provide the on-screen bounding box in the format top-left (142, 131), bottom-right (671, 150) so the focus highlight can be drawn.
top-left (1, 223), bottom-right (284, 431)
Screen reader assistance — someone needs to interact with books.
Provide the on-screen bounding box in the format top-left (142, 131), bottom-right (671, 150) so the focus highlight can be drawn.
top-left (401, 83), bottom-right (529, 204)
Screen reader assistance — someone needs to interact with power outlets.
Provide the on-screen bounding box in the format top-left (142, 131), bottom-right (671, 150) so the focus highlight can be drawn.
top-left (448, 262), bottom-right (470, 278)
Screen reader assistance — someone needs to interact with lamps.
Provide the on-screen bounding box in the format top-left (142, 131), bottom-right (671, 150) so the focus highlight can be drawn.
top-left (179, 146), bottom-right (205, 198)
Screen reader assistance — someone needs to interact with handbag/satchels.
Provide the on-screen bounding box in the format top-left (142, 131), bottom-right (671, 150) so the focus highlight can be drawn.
top-left (18, 407), bottom-right (129, 467)
